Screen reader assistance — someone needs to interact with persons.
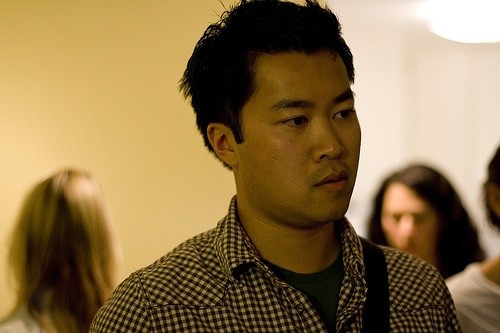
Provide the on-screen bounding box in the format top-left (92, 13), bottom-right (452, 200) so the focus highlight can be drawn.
top-left (1, 169), bottom-right (119, 333)
top-left (89, 0), bottom-right (463, 333)
top-left (367, 164), bottom-right (485, 279)
top-left (446, 148), bottom-right (500, 333)
